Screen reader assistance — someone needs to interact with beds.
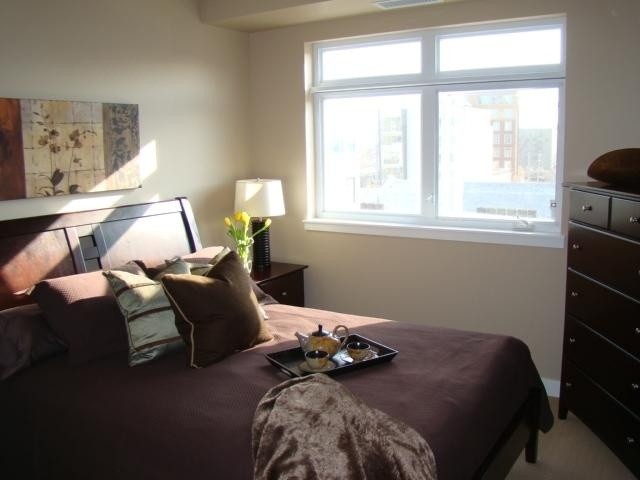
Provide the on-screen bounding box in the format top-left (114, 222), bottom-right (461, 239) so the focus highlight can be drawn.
top-left (1, 194), bottom-right (558, 479)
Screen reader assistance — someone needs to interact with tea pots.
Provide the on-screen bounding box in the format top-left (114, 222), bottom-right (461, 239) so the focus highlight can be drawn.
top-left (295, 324), bottom-right (348, 358)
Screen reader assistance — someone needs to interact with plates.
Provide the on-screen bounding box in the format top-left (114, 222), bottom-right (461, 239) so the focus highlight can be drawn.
top-left (342, 350), bottom-right (377, 364)
top-left (294, 359), bottom-right (339, 373)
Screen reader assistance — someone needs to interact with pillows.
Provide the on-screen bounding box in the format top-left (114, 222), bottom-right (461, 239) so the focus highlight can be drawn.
top-left (0, 301), bottom-right (68, 388)
top-left (100, 256), bottom-right (193, 369)
top-left (163, 244), bottom-right (269, 322)
top-left (148, 243), bottom-right (280, 308)
top-left (159, 250), bottom-right (274, 370)
top-left (13, 257), bottom-right (153, 372)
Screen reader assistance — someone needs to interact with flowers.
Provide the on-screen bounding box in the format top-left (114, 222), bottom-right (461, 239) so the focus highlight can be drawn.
top-left (222, 209), bottom-right (273, 263)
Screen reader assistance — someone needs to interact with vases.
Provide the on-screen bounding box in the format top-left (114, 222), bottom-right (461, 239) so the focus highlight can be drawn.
top-left (234, 237), bottom-right (254, 276)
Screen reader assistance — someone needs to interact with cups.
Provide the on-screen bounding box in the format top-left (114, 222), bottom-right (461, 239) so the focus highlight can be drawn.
top-left (304, 350), bottom-right (329, 369)
top-left (346, 342), bottom-right (372, 362)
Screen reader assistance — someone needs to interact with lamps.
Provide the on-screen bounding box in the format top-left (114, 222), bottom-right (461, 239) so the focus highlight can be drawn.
top-left (230, 176), bottom-right (289, 272)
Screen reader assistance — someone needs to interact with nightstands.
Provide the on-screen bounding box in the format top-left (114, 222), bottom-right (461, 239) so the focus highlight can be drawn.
top-left (245, 255), bottom-right (311, 309)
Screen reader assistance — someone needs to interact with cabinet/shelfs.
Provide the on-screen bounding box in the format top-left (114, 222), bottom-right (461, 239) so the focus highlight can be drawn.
top-left (556, 178), bottom-right (639, 480)
